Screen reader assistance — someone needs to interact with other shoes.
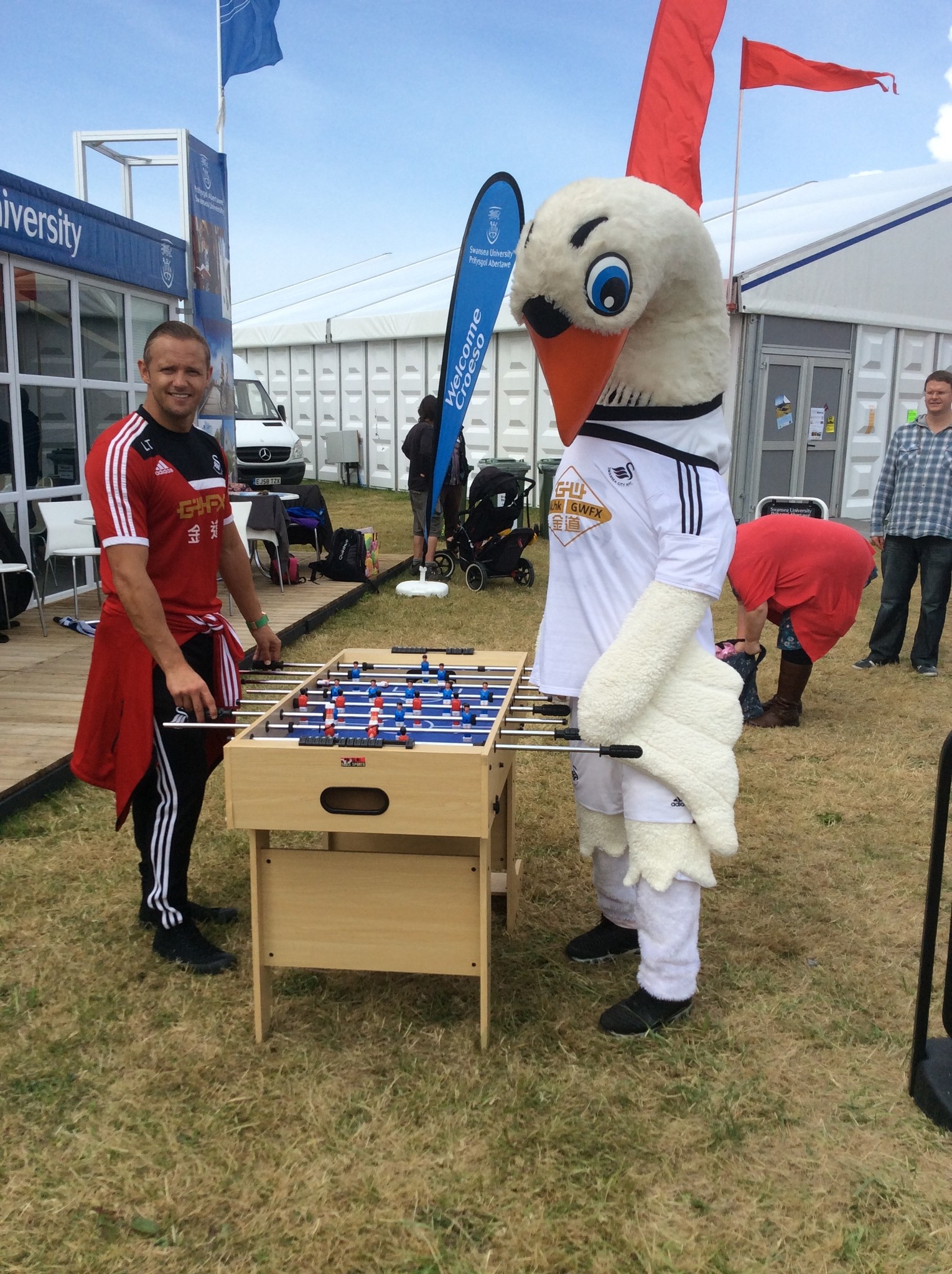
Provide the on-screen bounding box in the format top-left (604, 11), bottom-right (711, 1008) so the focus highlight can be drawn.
top-left (0, 620), bottom-right (19, 629)
top-left (0, 633), bottom-right (9, 643)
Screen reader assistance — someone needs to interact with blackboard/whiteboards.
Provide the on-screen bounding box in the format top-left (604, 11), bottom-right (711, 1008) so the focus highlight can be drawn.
top-left (754, 496), bottom-right (829, 519)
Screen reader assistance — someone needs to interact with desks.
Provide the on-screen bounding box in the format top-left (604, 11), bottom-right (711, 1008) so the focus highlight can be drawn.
top-left (229, 490), bottom-right (300, 581)
top-left (74, 518), bottom-right (96, 525)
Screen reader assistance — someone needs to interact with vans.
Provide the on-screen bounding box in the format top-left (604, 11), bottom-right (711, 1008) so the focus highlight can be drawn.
top-left (232, 353), bottom-right (306, 489)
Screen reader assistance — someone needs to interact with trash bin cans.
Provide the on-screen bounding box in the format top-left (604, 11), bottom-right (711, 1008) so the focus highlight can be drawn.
top-left (477, 457), bottom-right (530, 531)
top-left (533, 458), bottom-right (562, 538)
top-left (438, 464), bottom-right (475, 542)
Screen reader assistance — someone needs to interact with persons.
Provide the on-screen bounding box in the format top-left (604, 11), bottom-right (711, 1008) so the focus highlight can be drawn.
top-left (852, 370), bottom-right (952, 677)
top-left (84, 321), bottom-right (283, 971)
top-left (8, 388), bottom-right (42, 550)
top-left (727, 513), bottom-right (879, 729)
top-left (402, 394), bottom-right (469, 583)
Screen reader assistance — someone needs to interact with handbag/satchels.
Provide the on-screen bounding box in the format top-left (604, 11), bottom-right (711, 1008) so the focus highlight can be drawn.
top-left (715, 637), bottom-right (765, 720)
top-left (270, 553), bottom-right (298, 584)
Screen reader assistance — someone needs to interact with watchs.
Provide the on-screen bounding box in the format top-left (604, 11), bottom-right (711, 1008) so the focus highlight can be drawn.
top-left (245, 612), bottom-right (269, 630)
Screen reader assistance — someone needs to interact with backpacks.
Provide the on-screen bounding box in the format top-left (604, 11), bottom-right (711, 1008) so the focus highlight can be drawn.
top-left (326, 528), bottom-right (366, 582)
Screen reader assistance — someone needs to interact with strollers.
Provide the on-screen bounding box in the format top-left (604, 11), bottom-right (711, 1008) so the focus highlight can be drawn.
top-left (430, 466), bottom-right (537, 592)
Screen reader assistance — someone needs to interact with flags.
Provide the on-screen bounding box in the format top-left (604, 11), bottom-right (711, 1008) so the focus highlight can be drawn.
top-left (218, 0), bottom-right (283, 96)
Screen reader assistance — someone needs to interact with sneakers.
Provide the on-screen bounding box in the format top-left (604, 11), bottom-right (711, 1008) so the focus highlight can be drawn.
top-left (153, 921), bottom-right (236, 972)
top-left (410, 563), bottom-right (422, 575)
top-left (916, 662), bottom-right (938, 677)
top-left (566, 915), bottom-right (641, 962)
top-left (140, 896), bottom-right (236, 925)
top-left (853, 658), bottom-right (887, 670)
top-left (424, 566), bottom-right (447, 581)
top-left (598, 988), bottom-right (694, 1039)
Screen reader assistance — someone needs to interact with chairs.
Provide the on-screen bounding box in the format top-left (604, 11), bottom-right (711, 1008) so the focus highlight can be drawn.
top-left (0, 512), bottom-right (48, 636)
top-left (272, 484), bottom-right (338, 560)
top-left (248, 493), bottom-right (287, 592)
top-left (37, 500), bottom-right (104, 619)
top-left (219, 500), bottom-right (252, 616)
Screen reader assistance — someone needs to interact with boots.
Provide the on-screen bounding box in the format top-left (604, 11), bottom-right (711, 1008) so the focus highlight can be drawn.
top-left (750, 658), bottom-right (814, 728)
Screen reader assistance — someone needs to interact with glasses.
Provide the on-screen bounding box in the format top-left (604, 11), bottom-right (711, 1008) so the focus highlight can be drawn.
top-left (923, 390), bottom-right (952, 396)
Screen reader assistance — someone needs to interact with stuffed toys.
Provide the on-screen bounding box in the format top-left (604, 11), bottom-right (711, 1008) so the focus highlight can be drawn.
top-left (507, 178), bottom-right (744, 1038)
